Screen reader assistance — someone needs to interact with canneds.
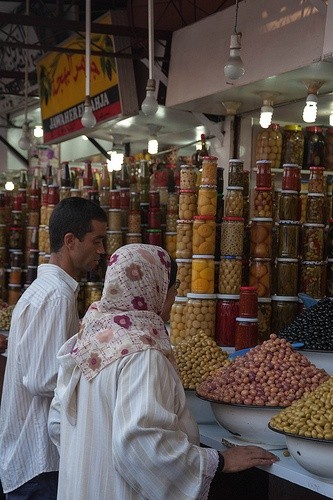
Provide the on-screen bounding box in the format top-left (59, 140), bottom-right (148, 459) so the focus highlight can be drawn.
top-left (0, 124), bottom-right (333, 351)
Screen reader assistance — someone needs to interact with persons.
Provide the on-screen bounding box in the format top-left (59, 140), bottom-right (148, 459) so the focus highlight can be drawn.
top-left (47, 243), bottom-right (281, 500)
top-left (0, 197), bottom-right (109, 500)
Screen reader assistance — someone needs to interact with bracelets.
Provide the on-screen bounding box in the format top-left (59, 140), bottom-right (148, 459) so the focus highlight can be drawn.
top-left (217, 453), bottom-right (224, 472)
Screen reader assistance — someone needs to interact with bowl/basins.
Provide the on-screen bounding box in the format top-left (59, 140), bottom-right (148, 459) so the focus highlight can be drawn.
top-left (266, 421), bottom-right (333, 484)
top-left (293, 348), bottom-right (333, 376)
top-left (194, 391), bottom-right (288, 446)
top-left (183, 387), bottom-right (215, 425)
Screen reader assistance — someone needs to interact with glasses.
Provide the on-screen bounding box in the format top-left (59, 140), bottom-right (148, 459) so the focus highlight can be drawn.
top-left (171, 278), bottom-right (181, 289)
